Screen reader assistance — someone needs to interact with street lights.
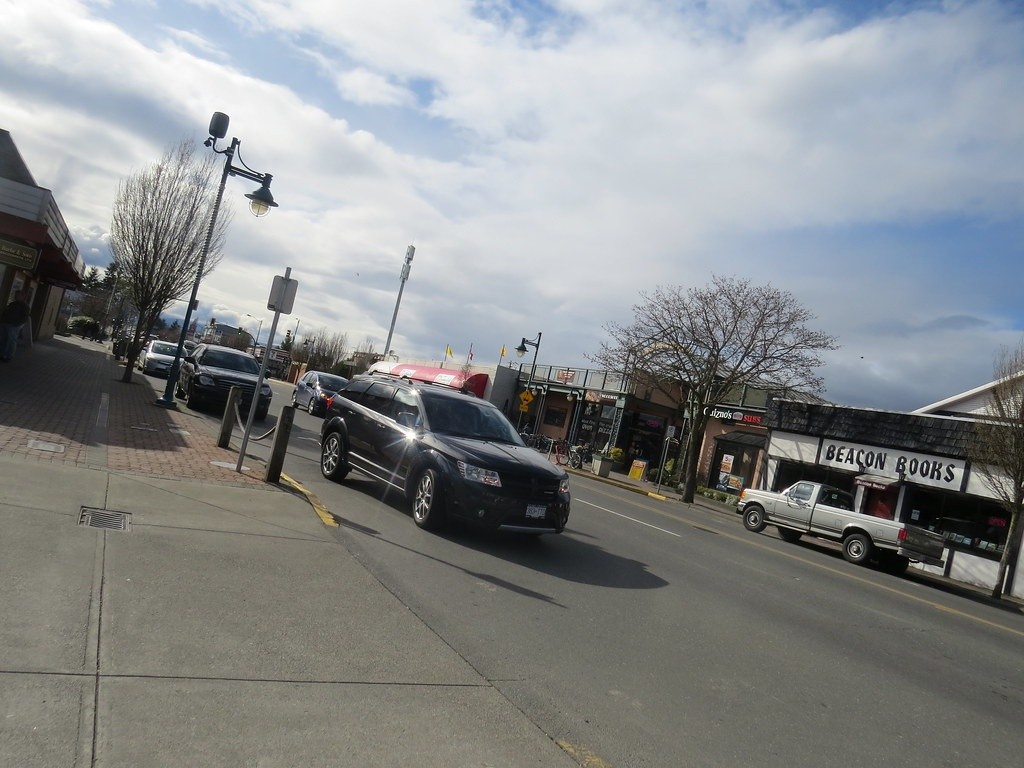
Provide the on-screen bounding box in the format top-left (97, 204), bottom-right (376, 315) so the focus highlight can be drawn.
top-left (154, 111), bottom-right (278, 404)
top-left (247, 314), bottom-right (263, 356)
top-left (292, 317), bottom-right (301, 342)
top-left (514, 331), bottom-right (543, 431)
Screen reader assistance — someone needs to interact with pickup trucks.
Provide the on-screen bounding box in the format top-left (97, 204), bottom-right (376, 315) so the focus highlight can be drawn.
top-left (736, 479), bottom-right (947, 578)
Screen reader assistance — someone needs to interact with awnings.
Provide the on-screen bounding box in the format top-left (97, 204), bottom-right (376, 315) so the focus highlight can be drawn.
top-left (854, 474), bottom-right (900, 491)
top-left (713, 431), bottom-right (766, 450)
top-left (368, 361), bottom-right (488, 396)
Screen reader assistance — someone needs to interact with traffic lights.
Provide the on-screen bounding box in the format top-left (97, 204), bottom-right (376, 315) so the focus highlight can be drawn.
top-left (238, 327), bottom-right (242, 335)
top-left (210, 318), bottom-right (215, 326)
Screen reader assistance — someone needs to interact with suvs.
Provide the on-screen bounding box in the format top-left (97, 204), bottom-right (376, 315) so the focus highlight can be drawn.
top-left (319, 370), bottom-right (571, 536)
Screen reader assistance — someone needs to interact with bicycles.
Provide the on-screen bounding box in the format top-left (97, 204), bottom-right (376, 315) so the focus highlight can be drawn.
top-left (519, 429), bottom-right (597, 470)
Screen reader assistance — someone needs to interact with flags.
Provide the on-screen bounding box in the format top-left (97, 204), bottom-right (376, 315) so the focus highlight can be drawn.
top-left (501, 347), bottom-right (506, 356)
top-left (447, 347), bottom-right (453, 357)
top-left (470, 352), bottom-right (473, 360)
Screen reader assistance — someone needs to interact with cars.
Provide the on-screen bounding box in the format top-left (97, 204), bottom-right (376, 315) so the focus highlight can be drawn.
top-left (124, 328), bottom-right (198, 357)
top-left (138, 339), bottom-right (188, 376)
top-left (292, 370), bottom-right (351, 418)
top-left (174, 342), bottom-right (272, 423)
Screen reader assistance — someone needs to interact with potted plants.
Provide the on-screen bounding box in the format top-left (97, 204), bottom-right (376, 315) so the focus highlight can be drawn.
top-left (607, 447), bottom-right (626, 472)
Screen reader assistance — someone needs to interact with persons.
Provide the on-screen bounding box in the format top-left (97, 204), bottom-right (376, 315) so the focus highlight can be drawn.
top-left (0, 291), bottom-right (30, 363)
top-left (82, 320), bottom-right (99, 341)
top-left (523, 423), bottom-right (531, 435)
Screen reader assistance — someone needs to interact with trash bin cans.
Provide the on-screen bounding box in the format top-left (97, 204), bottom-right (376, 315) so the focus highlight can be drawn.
top-left (112, 341), bottom-right (119, 353)
top-left (590, 454), bottom-right (614, 478)
